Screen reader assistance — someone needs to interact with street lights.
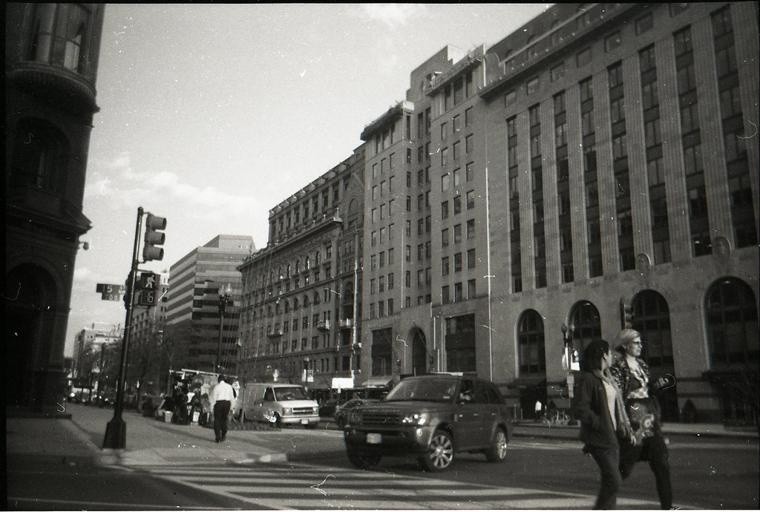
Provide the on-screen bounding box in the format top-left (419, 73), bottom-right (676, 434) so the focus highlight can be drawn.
top-left (217, 282), bottom-right (234, 371)
top-left (562, 322), bottom-right (578, 426)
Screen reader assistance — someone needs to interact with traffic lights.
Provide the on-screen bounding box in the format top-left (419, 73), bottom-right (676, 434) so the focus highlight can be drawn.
top-left (143, 213), bottom-right (166, 259)
top-left (139, 272), bottom-right (158, 290)
top-left (622, 299), bottom-right (633, 328)
top-left (138, 290), bottom-right (158, 305)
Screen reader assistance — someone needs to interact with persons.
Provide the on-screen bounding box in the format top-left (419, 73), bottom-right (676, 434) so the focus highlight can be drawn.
top-left (535, 399), bottom-right (542, 420)
top-left (574, 329), bottom-right (681, 510)
top-left (213, 375), bottom-right (234, 443)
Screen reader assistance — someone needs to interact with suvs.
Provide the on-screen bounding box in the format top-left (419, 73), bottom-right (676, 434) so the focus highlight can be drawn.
top-left (344, 369), bottom-right (513, 473)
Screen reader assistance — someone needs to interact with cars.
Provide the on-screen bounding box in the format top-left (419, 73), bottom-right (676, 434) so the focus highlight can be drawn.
top-left (67, 369), bottom-right (322, 429)
top-left (332, 398), bottom-right (380, 431)
